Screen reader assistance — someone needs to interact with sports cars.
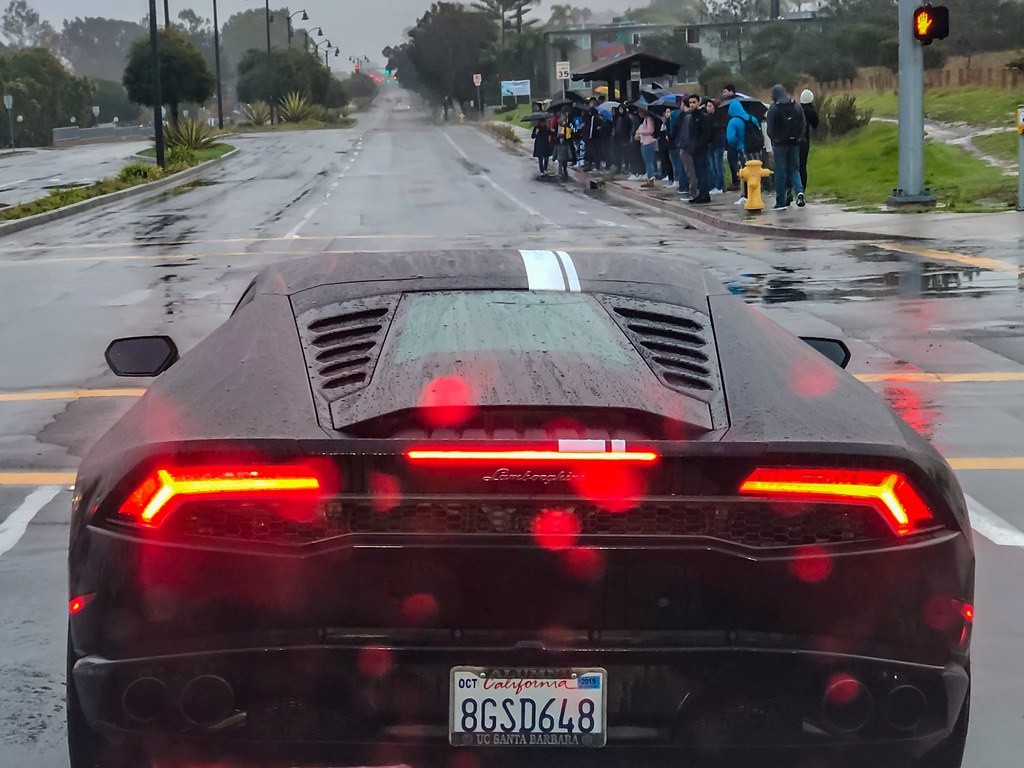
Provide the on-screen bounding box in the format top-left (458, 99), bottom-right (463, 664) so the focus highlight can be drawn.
top-left (69, 247), bottom-right (980, 768)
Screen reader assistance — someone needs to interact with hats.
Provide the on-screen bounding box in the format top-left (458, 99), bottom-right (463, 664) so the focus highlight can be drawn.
top-left (682, 95), bottom-right (690, 108)
top-left (708, 99), bottom-right (721, 110)
top-left (800, 89), bottom-right (814, 104)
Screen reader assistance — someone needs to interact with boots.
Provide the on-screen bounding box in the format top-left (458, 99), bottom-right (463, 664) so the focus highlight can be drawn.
top-left (640, 177), bottom-right (655, 187)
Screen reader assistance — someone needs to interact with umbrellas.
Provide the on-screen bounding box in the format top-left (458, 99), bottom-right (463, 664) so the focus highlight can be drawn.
top-left (520, 112), bottom-right (552, 123)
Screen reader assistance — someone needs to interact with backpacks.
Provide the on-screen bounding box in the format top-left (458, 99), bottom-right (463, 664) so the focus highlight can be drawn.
top-left (731, 113), bottom-right (765, 154)
top-left (774, 99), bottom-right (803, 145)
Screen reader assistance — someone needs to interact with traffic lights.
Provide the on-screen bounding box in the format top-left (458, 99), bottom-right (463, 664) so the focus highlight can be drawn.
top-left (913, 4), bottom-right (951, 46)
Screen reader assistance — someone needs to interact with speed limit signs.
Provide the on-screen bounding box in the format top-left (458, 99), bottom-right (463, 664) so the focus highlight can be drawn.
top-left (556, 61), bottom-right (570, 79)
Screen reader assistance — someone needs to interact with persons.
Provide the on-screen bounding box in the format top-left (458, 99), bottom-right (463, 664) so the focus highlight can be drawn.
top-left (531, 84), bottom-right (819, 211)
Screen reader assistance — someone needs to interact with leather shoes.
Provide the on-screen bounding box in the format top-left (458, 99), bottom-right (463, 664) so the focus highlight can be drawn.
top-left (689, 194), bottom-right (711, 203)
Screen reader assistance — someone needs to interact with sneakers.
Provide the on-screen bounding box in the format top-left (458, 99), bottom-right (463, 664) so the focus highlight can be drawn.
top-left (785, 194), bottom-right (794, 207)
top-left (709, 187), bottom-right (723, 195)
top-left (662, 174), bottom-right (696, 200)
top-left (795, 192), bottom-right (805, 207)
top-left (733, 196), bottom-right (748, 205)
top-left (770, 203), bottom-right (786, 210)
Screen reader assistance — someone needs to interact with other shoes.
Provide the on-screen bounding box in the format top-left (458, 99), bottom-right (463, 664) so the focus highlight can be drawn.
top-left (726, 185), bottom-right (741, 191)
top-left (539, 161), bottom-right (646, 182)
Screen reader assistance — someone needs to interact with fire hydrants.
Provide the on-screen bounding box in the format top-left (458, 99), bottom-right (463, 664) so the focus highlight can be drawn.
top-left (737, 159), bottom-right (775, 217)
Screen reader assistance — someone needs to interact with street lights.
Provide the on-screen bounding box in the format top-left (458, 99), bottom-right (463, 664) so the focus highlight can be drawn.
top-left (266, 4), bottom-right (373, 78)
